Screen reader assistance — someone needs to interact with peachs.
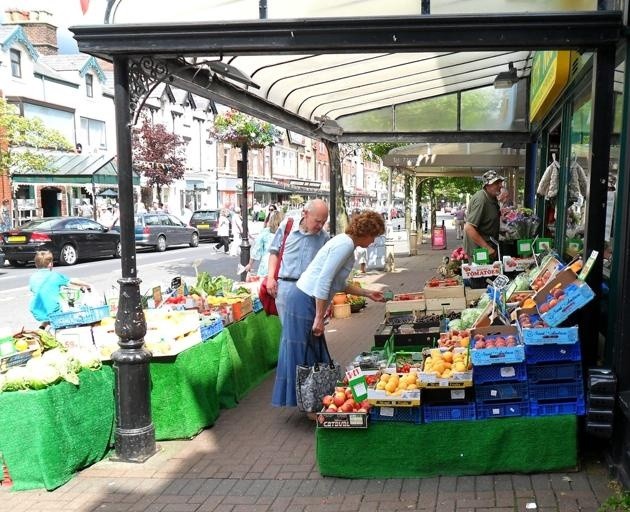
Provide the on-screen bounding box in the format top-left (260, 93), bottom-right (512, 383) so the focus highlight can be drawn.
top-left (510, 270), bottom-right (565, 329)
top-left (473, 333), bottom-right (518, 358)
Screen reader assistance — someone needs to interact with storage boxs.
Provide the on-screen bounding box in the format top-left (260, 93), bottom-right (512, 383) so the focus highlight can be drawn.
top-left (189, 294), bottom-right (253, 326)
top-left (382, 277), bottom-right (495, 315)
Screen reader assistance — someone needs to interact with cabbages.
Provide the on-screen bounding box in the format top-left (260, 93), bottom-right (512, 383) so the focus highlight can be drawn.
top-left (0, 349), bottom-right (103, 392)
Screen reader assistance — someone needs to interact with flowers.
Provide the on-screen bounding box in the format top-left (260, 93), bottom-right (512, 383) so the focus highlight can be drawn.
top-left (500, 205), bottom-right (540, 239)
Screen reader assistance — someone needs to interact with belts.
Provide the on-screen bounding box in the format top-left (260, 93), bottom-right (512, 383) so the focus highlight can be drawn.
top-left (278, 277), bottom-right (298, 281)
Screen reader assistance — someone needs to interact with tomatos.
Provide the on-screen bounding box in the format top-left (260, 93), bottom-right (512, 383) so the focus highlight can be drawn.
top-left (167, 296), bottom-right (187, 304)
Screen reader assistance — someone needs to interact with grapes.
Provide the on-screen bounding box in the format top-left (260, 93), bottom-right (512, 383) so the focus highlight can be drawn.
top-left (386, 312), bottom-right (460, 328)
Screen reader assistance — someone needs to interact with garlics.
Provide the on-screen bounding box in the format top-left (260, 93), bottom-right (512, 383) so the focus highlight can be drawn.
top-left (536, 164), bottom-right (559, 198)
top-left (568, 162), bottom-right (587, 200)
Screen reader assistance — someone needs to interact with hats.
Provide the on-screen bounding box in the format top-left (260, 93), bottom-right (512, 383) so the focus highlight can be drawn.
top-left (482, 170), bottom-right (508, 188)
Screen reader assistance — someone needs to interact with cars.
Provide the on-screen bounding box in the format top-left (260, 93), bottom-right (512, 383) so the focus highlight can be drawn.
top-left (188, 207), bottom-right (234, 244)
top-left (0, 247), bottom-right (6, 268)
top-left (110, 210), bottom-right (200, 252)
top-left (0, 213), bottom-right (122, 268)
top-left (379, 208), bottom-right (406, 230)
top-left (444, 207), bottom-right (451, 213)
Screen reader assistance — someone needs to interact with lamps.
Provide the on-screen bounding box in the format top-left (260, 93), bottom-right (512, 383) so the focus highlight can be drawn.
top-left (194, 60), bottom-right (262, 90)
top-left (492, 68), bottom-right (531, 89)
top-left (313, 119), bottom-right (344, 136)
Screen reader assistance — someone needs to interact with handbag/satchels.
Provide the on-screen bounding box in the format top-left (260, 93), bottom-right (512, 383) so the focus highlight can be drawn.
top-left (259, 275), bottom-right (278, 315)
top-left (294, 325), bottom-right (342, 412)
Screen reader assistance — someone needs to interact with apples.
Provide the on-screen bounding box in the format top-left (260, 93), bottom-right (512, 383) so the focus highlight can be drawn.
top-left (322, 387), bottom-right (371, 418)
top-left (422, 329), bottom-right (471, 379)
top-left (16, 339), bottom-right (28, 352)
top-left (451, 247), bottom-right (468, 260)
top-left (99, 309), bottom-right (196, 355)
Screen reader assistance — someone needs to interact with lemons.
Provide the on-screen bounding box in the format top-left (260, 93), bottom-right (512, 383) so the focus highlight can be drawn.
top-left (207, 296), bottom-right (243, 305)
top-left (376, 372), bottom-right (419, 408)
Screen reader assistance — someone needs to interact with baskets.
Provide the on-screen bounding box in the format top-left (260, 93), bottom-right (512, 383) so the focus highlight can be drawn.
top-left (0, 348), bottom-right (41, 373)
top-left (421, 401), bottom-right (476, 421)
top-left (49, 305), bottom-right (110, 329)
top-left (198, 318), bottom-right (225, 341)
top-left (253, 299), bottom-right (264, 312)
top-left (473, 343), bottom-right (586, 420)
top-left (371, 405), bottom-right (421, 422)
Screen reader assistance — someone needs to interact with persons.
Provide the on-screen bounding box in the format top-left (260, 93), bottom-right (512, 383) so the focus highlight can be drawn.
top-left (26, 250), bottom-right (94, 331)
top-left (74, 189), bottom-right (212, 230)
top-left (462, 169), bottom-right (506, 264)
top-left (212, 198), bottom-right (466, 273)
top-left (270, 211), bottom-right (388, 422)
top-left (265, 198), bottom-right (332, 326)
top-left (1, 196), bottom-right (13, 234)
